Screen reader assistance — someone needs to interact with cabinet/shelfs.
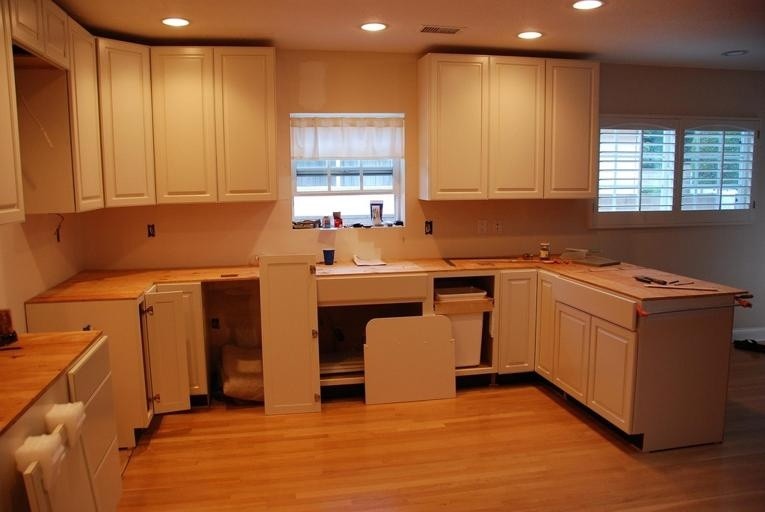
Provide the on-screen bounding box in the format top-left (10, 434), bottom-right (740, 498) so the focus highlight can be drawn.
top-left (430, 54), bottom-right (487, 200)
top-left (158, 282), bottom-right (209, 396)
top-left (499, 268), bottom-right (535, 374)
top-left (545, 58), bottom-right (602, 199)
top-left (96, 38), bottom-right (156, 209)
top-left (555, 276), bottom-right (688, 436)
top-left (0, 7), bottom-right (17, 207)
top-left (318, 274), bottom-right (428, 304)
top-left (136, 291), bottom-right (191, 427)
top-left (487, 56), bottom-right (547, 200)
top-left (24, 424), bottom-right (100, 512)
top-left (67, 336), bottom-right (123, 512)
top-left (214, 47), bottom-right (276, 202)
top-left (144, 285), bottom-right (157, 294)
top-left (69, 26), bottom-right (104, 214)
top-left (151, 47), bottom-right (219, 205)
top-left (537, 267), bottom-right (555, 381)
top-left (9, 0), bottom-right (70, 70)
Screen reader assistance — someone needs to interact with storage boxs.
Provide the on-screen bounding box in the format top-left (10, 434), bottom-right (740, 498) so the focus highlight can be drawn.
top-left (434, 287), bottom-right (487, 368)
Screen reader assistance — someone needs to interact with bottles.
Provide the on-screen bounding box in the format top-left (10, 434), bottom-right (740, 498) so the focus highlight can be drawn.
top-left (539, 243), bottom-right (550, 260)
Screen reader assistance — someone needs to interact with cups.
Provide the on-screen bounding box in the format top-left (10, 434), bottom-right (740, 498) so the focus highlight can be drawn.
top-left (323, 248), bottom-right (335, 265)
